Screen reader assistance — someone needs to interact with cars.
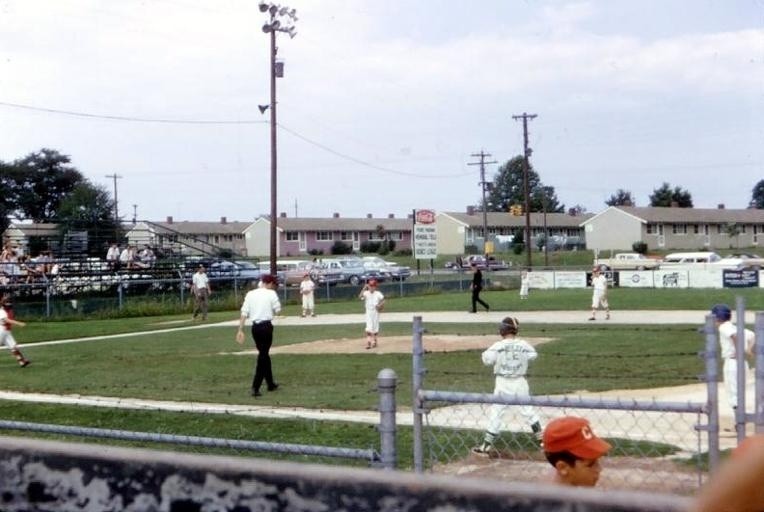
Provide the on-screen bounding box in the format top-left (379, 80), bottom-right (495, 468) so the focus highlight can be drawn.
top-left (711, 252), bottom-right (764, 271)
top-left (592, 252), bottom-right (662, 271)
top-left (495, 235), bottom-right (526, 249)
top-left (175, 257), bottom-right (411, 289)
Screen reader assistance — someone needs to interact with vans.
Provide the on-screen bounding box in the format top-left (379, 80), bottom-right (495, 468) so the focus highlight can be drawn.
top-left (656, 253), bottom-right (721, 264)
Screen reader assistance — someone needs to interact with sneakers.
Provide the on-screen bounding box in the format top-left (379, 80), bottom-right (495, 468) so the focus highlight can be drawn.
top-left (472, 445), bottom-right (490, 459)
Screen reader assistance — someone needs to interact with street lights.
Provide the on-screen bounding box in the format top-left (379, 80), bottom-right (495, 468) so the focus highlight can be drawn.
top-left (257, 0), bottom-right (298, 297)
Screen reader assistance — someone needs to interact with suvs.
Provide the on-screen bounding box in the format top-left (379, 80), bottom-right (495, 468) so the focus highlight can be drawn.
top-left (50, 261), bottom-right (153, 298)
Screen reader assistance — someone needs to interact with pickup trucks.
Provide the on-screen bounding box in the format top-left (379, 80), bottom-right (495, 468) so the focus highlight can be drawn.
top-left (444, 254), bottom-right (512, 272)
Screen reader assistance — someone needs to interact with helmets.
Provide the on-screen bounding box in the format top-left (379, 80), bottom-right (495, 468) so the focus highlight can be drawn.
top-left (500, 316), bottom-right (520, 336)
top-left (711, 303), bottom-right (732, 322)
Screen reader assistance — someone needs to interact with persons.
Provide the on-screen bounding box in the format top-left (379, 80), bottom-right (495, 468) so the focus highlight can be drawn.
top-left (1, 290), bottom-right (31, 368)
top-left (235, 271), bottom-right (285, 399)
top-left (588, 266), bottom-right (610, 320)
top-left (107, 242), bottom-right (157, 276)
top-left (712, 306), bottom-right (758, 427)
top-left (299, 274), bottom-right (317, 318)
top-left (470, 262), bottom-right (490, 312)
top-left (474, 318), bottom-right (548, 458)
top-left (541, 411), bottom-right (615, 490)
top-left (192, 266), bottom-right (213, 320)
top-left (1, 237), bottom-right (61, 295)
top-left (693, 430), bottom-right (763, 512)
top-left (359, 277), bottom-right (386, 351)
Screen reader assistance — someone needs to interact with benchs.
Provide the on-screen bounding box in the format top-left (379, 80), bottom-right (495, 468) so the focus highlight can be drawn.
top-left (25, 250), bottom-right (233, 279)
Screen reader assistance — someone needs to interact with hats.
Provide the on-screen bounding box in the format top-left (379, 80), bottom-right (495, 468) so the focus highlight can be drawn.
top-left (540, 416), bottom-right (612, 460)
top-left (368, 280), bottom-right (377, 288)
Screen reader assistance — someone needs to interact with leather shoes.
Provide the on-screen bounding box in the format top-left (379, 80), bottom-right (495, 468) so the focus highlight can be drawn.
top-left (268, 383), bottom-right (280, 391)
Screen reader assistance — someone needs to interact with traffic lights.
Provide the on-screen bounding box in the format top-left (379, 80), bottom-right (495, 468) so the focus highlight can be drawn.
top-left (513, 209), bottom-right (517, 216)
top-left (517, 205), bottom-right (521, 216)
top-left (509, 206), bottom-right (515, 216)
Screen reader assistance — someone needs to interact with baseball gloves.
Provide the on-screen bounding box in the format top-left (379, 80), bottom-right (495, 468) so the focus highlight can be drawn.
top-left (376, 303), bottom-right (383, 310)
